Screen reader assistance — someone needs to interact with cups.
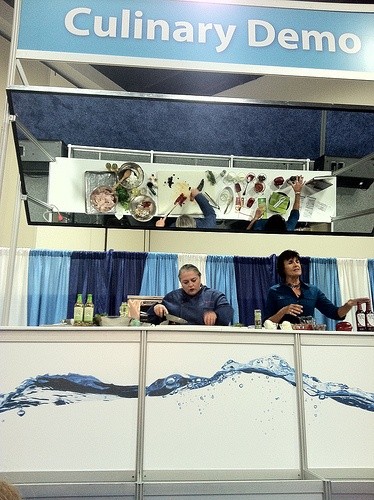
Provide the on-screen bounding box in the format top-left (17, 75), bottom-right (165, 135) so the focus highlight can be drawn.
top-left (300, 316), bottom-right (315, 329)
top-left (120, 302), bottom-right (129, 318)
top-left (254, 309), bottom-right (262, 329)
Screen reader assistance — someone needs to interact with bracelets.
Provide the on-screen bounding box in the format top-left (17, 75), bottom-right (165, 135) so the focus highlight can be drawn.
top-left (296, 191), bottom-right (300, 194)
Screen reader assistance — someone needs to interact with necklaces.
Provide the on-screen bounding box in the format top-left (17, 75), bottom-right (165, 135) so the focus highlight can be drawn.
top-left (289, 282), bottom-right (299, 288)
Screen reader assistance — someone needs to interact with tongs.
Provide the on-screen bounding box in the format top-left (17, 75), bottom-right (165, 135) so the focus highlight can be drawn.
top-left (205, 192), bottom-right (220, 210)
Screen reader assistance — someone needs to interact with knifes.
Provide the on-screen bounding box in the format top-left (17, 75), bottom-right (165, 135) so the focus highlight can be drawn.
top-left (162, 203), bottom-right (180, 219)
top-left (164, 312), bottom-right (188, 323)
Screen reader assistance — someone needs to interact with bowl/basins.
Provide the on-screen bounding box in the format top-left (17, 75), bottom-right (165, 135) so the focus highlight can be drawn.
top-left (90, 185), bottom-right (118, 213)
top-left (223, 171), bottom-right (267, 193)
top-left (269, 192), bottom-right (291, 213)
top-left (279, 324), bottom-right (294, 330)
top-left (129, 195), bottom-right (156, 222)
top-left (216, 186), bottom-right (234, 214)
top-left (295, 324), bottom-right (326, 331)
top-left (70, 319), bottom-right (74, 325)
top-left (263, 323), bottom-right (278, 330)
top-left (95, 317), bottom-right (133, 326)
top-left (116, 162), bottom-right (144, 189)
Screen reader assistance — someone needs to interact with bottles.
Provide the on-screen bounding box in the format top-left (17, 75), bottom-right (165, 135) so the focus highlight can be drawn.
top-left (84, 294), bottom-right (94, 323)
top-left (355, 302), bottom-right (367, 331)
top-left (365, 301), bottom-right (374, 331)
top-left (215, 170), bottom-right (226, 184)
top-left (74, 294), bottom-right (84, 324)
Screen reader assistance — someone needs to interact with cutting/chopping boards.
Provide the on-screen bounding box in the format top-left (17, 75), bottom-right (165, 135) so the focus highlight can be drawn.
top-left (157, 170), bottom-right (221, 214)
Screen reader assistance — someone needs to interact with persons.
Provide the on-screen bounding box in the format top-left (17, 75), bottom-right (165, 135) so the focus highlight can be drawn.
top-left (155, 188), bottom-right (216, 228)
top-left (247, 175), bottom-right (306, 231)
top-left (146, 263), bottom-right (235, 326)
top-left (266, 250), bottom-right (370, 326)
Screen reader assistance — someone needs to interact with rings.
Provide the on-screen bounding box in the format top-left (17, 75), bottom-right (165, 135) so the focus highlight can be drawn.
top-left (291, 309), bottom-right (293, 311)
top-left (290, 312), bottom-right (291, 314)
